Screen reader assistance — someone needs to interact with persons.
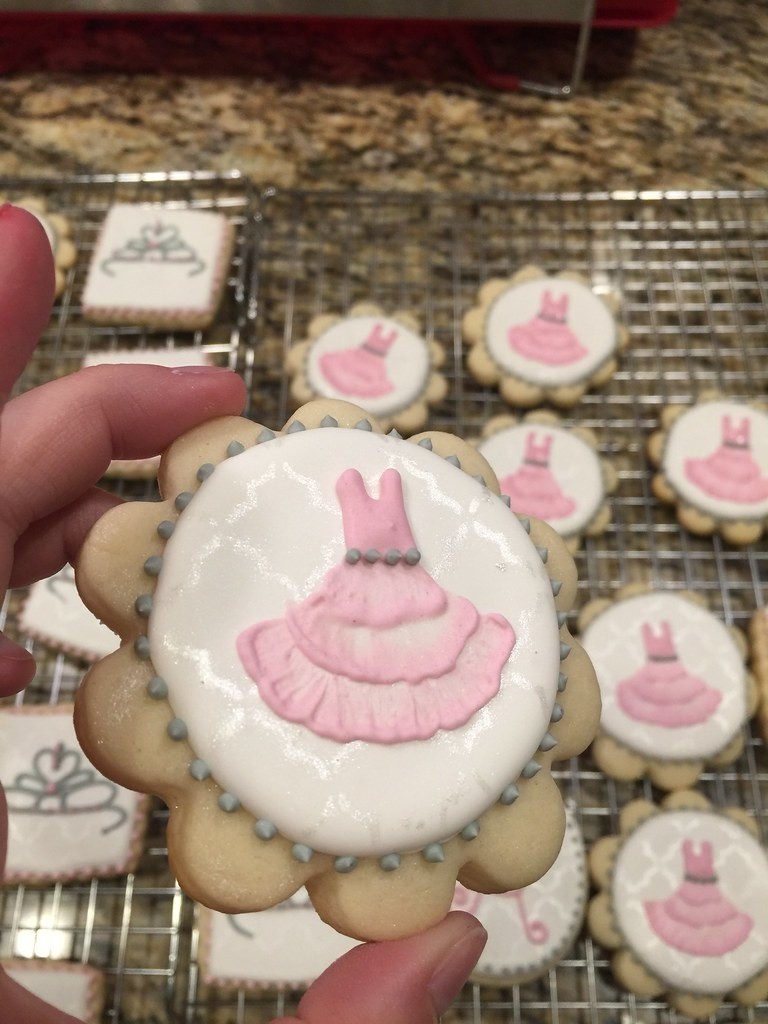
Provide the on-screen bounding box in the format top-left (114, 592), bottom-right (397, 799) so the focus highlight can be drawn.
top-left (4, 200), bottom-right (489, 1024)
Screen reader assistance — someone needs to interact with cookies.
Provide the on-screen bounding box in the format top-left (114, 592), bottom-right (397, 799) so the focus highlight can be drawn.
top-left (0, 194), bottom-right (768, 1017)
